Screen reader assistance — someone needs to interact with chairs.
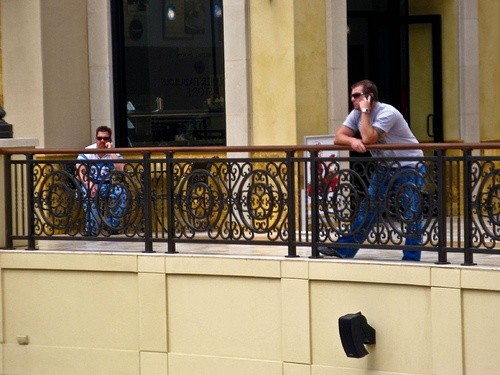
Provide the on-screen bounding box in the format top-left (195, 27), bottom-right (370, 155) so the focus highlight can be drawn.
top-left (60, 163), bottom-right (106, 235)
top-left (162, 156), bottom-right (220, 234)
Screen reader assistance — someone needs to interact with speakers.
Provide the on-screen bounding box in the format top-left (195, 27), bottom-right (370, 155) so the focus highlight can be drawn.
top-left (339, 312), bottom-right (375, 357)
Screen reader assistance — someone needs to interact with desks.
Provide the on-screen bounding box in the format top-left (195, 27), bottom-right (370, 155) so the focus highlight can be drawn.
top-left (126, 169), bottom-right (168, 235)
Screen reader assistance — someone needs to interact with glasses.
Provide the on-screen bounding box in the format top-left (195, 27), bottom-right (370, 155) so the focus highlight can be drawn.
top-left (351, 93), bottom-right (365, 98)
top-left (96, 137), bottom-right (111, 140)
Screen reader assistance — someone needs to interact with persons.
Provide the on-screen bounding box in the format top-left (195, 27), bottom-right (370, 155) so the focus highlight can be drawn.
top-left (74, 126), bottom-right (129, 237)
top-left (317, 79), bottom-right (427, 261)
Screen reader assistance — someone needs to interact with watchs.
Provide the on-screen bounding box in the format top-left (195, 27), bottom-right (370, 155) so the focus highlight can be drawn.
top-left (361, 108), bottom-right (370, 113)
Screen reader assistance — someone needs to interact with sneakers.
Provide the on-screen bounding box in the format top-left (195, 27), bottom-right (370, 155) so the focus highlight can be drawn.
top-left (317, 246), bottom-right (336, 256)
top-left (103, 225), bottom-right (118, 235)
